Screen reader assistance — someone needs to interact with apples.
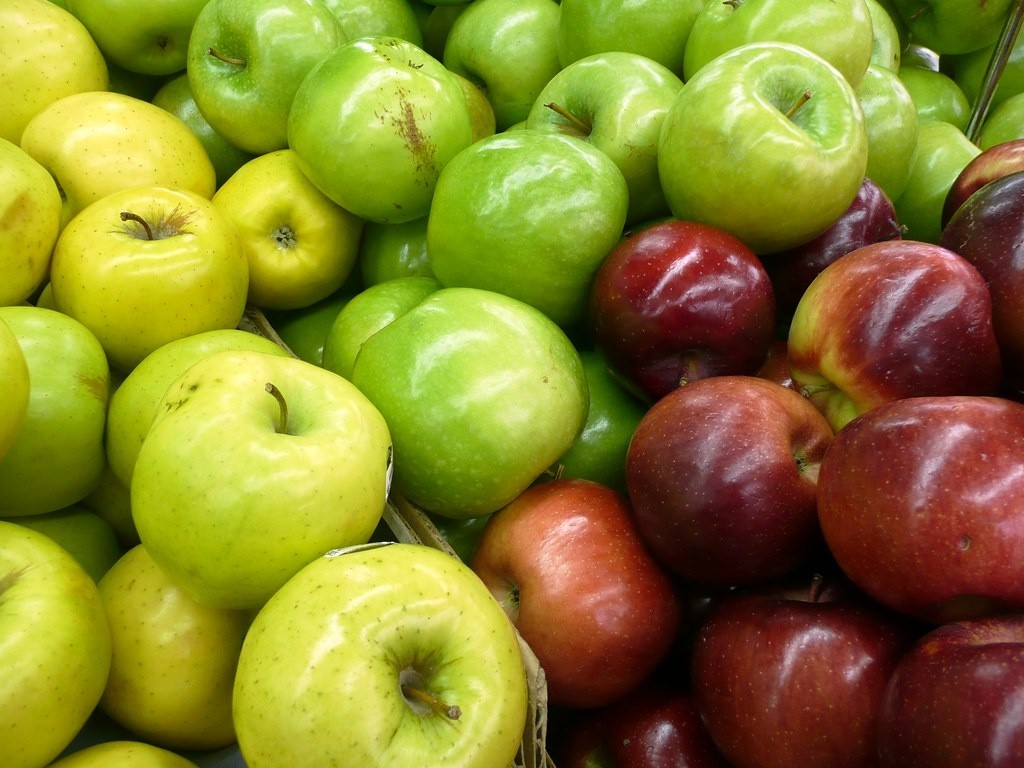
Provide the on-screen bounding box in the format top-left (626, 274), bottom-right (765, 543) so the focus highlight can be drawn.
top-left (0, 0), bottom-right (1024, 768)
